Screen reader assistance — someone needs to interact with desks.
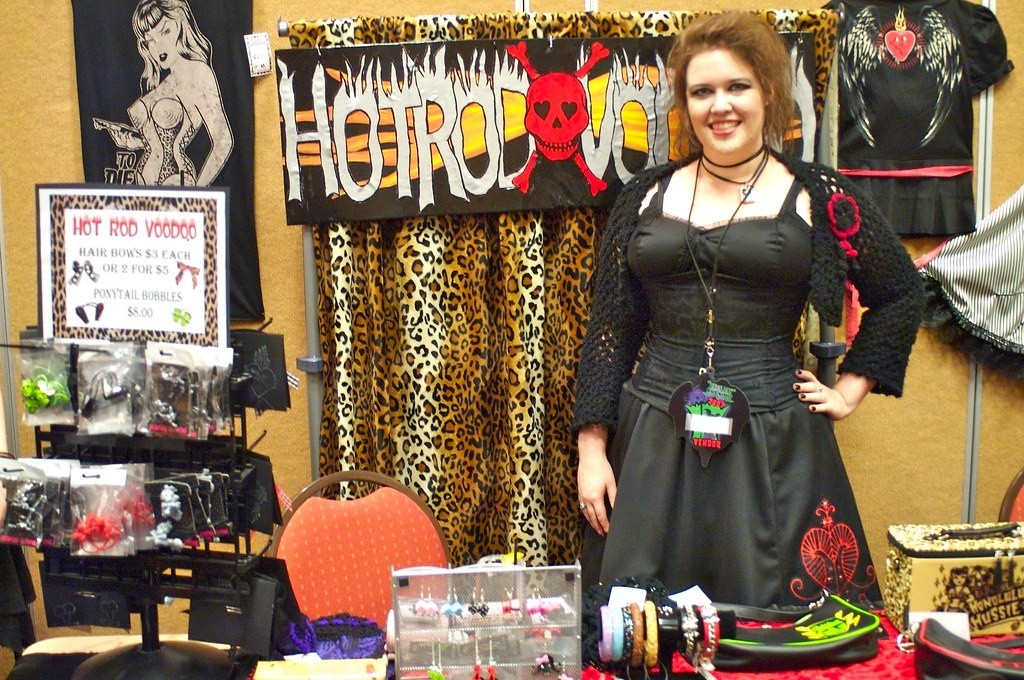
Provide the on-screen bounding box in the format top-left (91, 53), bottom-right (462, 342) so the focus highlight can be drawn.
top-left (5, 631), bottom-right (249, 680)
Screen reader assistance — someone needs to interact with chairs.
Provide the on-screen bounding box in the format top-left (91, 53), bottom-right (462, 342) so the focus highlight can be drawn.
top-left (268, 471), bottom-right (452, 630)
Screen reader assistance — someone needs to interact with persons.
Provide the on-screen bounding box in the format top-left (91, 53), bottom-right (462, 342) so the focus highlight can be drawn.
top-left (570, 12), bottom-right (925, 610)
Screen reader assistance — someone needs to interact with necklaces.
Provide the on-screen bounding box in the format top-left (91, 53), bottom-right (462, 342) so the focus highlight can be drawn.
top-left (667, 145), bottom-right (769, 469)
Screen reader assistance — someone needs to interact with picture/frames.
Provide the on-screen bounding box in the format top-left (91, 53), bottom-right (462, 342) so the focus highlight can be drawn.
top-left (34, 183), bottom-right (232, 348)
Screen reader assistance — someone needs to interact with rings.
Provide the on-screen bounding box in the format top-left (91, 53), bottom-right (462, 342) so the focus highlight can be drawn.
top-left (820, 388), bottom-right (822, 392)
top-left (580, 503), bottom-right (587, 509)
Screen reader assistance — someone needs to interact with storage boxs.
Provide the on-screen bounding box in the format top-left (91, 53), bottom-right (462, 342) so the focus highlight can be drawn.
top-left (885, 521), bottom-right (1024, 640)
top-left (389, 559), bottom-right (583, 680)
